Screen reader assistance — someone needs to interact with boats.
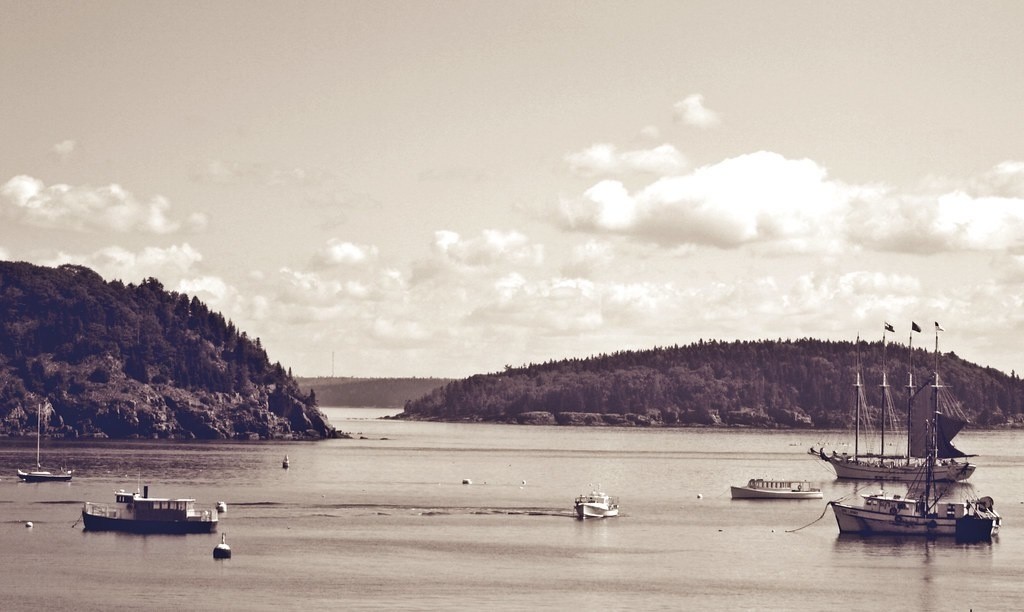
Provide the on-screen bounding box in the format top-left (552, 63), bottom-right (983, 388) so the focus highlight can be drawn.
top-left (829, 418), bottom-right (1002, 539)
top-left (730, 478), bottom-right (825, 501)
top-left (572, 483), bottom-right (623, 520)
top-left (78, 466), bottom-right (224, 532)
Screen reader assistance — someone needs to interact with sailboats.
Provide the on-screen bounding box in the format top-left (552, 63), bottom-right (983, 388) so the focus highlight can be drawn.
top-left (805, 322), bottom-right (978, 483)
top-left (16, 404), bottom-right (77, 482)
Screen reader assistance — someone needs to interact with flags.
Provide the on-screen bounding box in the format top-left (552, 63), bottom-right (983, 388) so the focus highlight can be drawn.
top-left (885, 322), bottom-right (895, 332)
top-left (935, 322), bottom-right (945, 331)
top-left (912, 321), bottom-right (921, 332)
